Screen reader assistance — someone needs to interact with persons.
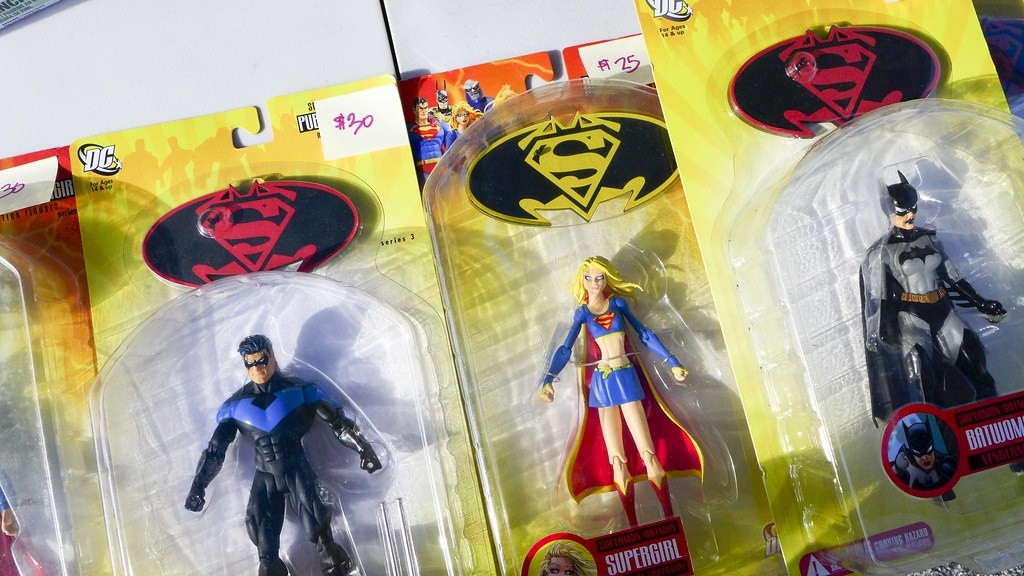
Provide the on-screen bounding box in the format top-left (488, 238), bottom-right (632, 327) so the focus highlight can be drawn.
top-left (539, 255), bottom-right (688, 528)
top-left (185, 334), bottom-right (382, 576)
top-left (859, 170), bottom-right (1024, 503)
